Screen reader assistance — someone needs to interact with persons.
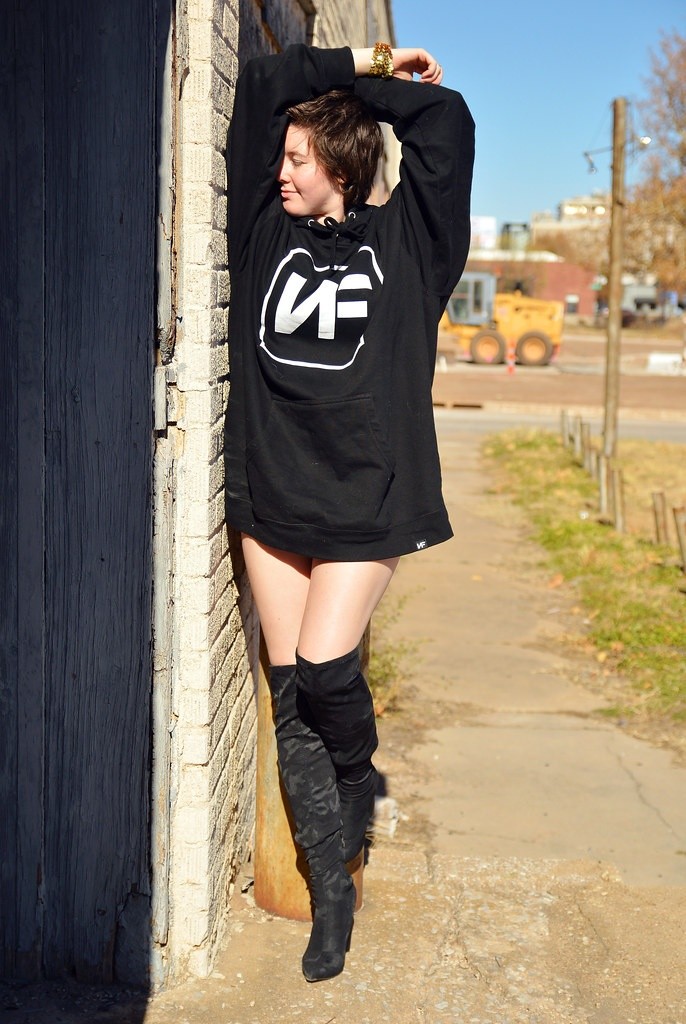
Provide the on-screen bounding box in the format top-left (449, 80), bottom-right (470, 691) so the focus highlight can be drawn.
top-left (227, 37), bottom-right (478, 985)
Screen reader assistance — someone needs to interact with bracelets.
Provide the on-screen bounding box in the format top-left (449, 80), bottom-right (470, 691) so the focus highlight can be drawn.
top-left (371, 41), bottom-right (395, 77)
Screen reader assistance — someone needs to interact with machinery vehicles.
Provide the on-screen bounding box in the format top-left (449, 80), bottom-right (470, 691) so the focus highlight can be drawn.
top-left (436, 272), bottom-right (567, 368)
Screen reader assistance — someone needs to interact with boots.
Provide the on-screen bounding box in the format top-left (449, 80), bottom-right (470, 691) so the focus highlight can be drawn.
top-left (263, 653), bottom-right (379, 980)
